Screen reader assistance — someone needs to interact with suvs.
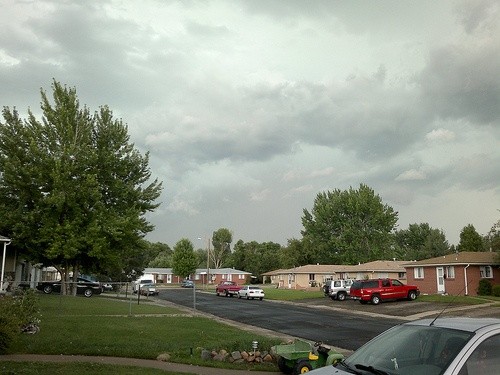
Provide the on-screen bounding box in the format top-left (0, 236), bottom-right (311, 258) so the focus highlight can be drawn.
top-left (215, 280), bottom-right (243, 297)
top-left (322, 279), bottom-right (361, 301)
top-left (349, 278), bottom-right (421, 305)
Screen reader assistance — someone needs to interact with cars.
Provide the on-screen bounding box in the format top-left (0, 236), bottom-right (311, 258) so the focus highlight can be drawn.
top-left (36, 276), bottom-right (112, 298)
top-left (299, 316), bottom-right (500, 375)
top-left (182, 280), bottom-right (194, 288)
top-left (138, 279), bottom-right (160, 296)
top-left (237, 286), bottom-right (265, 300)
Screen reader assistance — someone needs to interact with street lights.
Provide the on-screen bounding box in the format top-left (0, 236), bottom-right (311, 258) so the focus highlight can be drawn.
top-left (197, 237), bottom-right (211, 284)
